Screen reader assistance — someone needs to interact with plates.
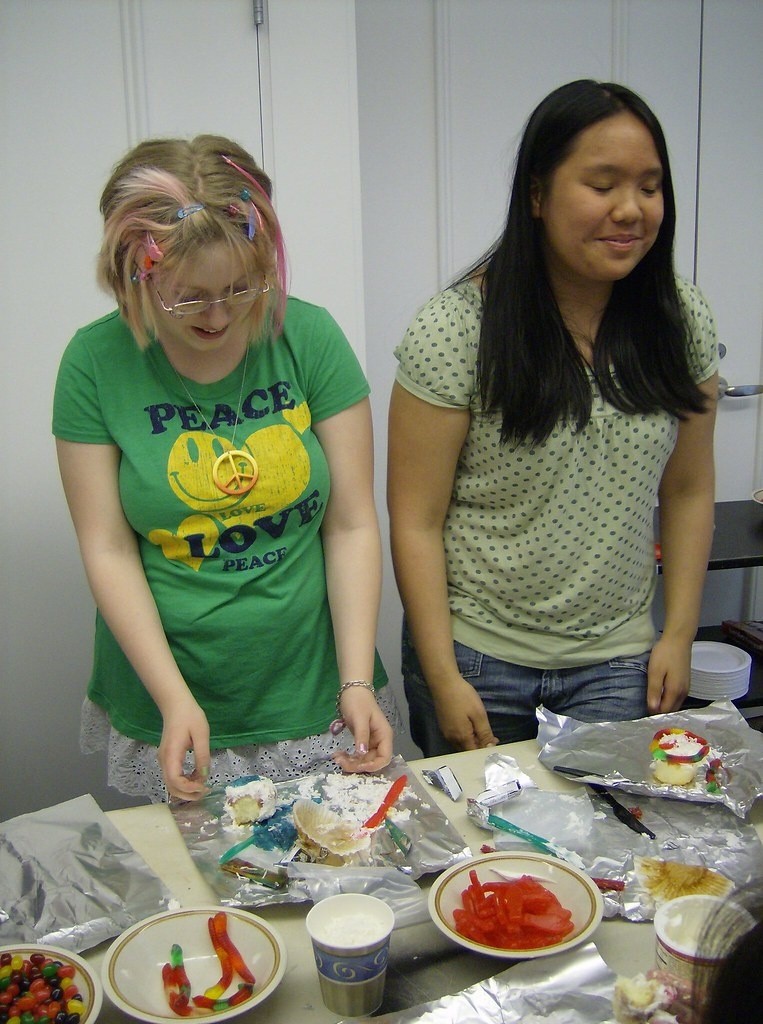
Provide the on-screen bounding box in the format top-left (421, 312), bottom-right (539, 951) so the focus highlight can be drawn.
top-left (688, 640), bottom-right (752, 701)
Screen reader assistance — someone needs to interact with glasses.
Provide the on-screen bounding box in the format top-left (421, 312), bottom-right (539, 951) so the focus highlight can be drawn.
top-left (146, 267), bottom-right (271, 313)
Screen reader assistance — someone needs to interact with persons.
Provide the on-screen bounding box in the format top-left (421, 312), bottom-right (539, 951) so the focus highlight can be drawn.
top-left (384, 79), bottom-right (725, 758)
top-left (51, 133), bottom-right (394, 804)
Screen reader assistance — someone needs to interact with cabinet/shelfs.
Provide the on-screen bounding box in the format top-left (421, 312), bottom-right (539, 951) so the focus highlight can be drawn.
top-left (653, 500), bottom-right (763, 719)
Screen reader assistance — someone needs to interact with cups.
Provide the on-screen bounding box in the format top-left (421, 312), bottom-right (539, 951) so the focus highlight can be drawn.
top-left (653, 894), bottom-right (758, 1001)
top-left (306, 893), bottom-right (395, 1019)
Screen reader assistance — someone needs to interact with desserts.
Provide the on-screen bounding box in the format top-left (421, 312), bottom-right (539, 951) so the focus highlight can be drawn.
top-left (611, 973), bottom-right (677, 1024)
top-left (649, 728), bottom-right (710, 786)
top-left (223, 775), bottom-right (276, 824)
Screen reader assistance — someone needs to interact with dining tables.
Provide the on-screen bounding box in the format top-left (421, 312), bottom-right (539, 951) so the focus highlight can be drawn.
top-left (83, 738), bottom-right (763, 1024)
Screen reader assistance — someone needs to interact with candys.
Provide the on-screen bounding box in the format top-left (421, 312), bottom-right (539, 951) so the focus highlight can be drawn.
top-left (705, 758), bottom-right (721, 794)
top-left (365, 775), bottom-right (407, 828)
top-left (452, 870), bottom-right (575, 948)
top-left (162, 912), bottom-right (256, 1017)
top-left (0, 952), bottom-right (86, 1024)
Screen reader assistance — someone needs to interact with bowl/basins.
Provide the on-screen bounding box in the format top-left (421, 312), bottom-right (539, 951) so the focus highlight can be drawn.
top-left (0, 944), bottom-right (103, 1024)
top-left (427, 851), bottom-right (604, 959)
top-left (101, 906), bottom-right (288, 1024)
top-left (752, 489), bottom-right (763, 506)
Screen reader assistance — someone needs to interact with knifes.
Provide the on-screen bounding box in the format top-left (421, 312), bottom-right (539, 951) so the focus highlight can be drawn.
top-left (589, 783), bottom-right (656, 840)
